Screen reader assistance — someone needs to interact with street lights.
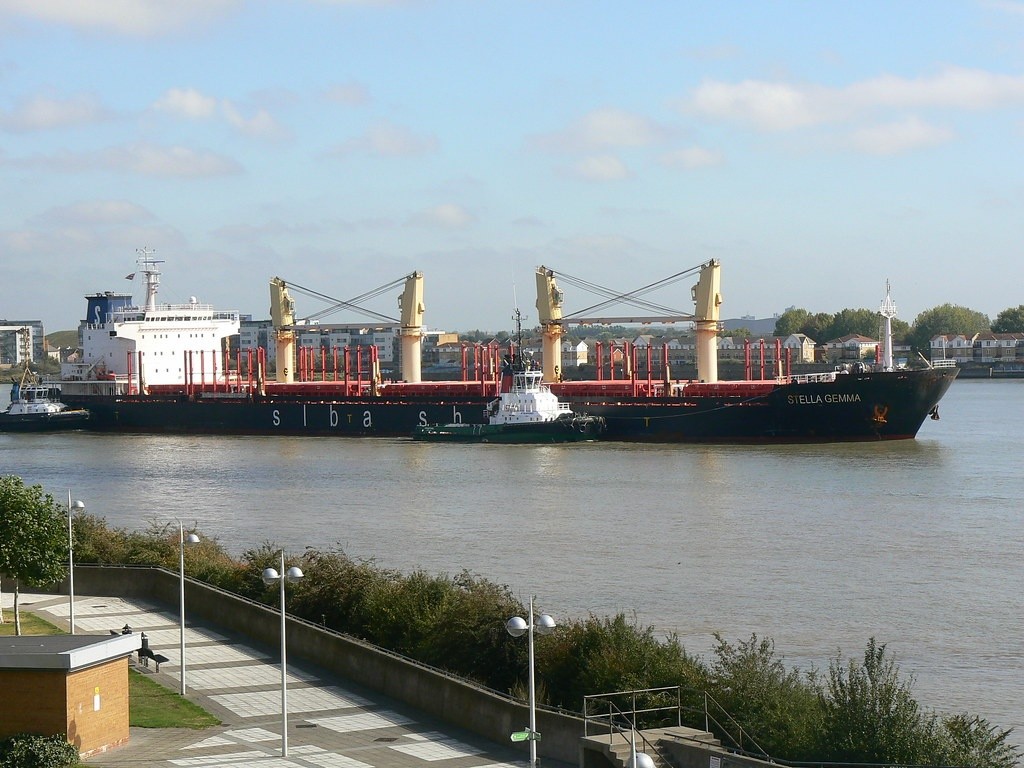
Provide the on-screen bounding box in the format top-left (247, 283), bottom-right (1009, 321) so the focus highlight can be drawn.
top-left (68, 489), bottom-right (84, 634)
top-left (505, 593), bottom-right (557, 768)
top-left (261, 549), bottom-right (305, 758)
top-left (177, 522), bottom-right (202, 696)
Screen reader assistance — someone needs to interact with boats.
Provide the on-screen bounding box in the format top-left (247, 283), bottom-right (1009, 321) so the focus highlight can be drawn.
top-left (413, 369), bottom-right (608, 444)
top-left (0, 385), bottom-right (90, 434)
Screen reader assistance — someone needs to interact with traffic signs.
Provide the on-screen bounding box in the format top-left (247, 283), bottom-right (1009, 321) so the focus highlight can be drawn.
top-left (525, 726), bottom-right (542, 742)
top-left (510, 731), bottom-right (531, 743)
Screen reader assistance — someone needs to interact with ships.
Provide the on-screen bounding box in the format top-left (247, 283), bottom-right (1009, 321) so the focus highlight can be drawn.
top-left (58, 247), bottom-right (964, 444)
